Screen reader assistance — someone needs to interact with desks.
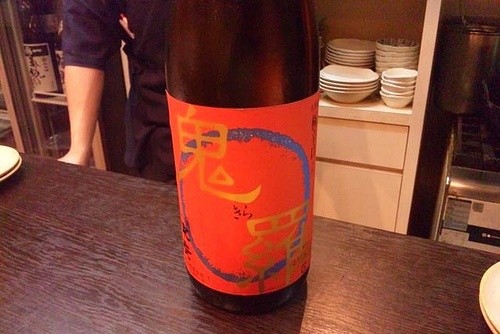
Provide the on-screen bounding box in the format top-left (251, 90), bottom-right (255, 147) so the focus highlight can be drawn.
top-left (0, 154), bottom-right (500, 334)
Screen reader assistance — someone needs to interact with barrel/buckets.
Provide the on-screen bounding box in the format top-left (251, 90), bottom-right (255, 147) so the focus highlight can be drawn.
top-left (432, 23), bottom-right (500, 115)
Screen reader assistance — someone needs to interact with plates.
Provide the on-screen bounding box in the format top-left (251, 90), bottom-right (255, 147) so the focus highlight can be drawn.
top-left (478, 261), bottom-right (500, 334)
top-left (0, 145), bottom-right (22, 182)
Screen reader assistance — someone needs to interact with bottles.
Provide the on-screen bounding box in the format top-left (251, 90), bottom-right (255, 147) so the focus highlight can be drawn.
top-left (16, 0), bottom-right (66, 101)
top-left (164, 0), bottom-right (319, 315)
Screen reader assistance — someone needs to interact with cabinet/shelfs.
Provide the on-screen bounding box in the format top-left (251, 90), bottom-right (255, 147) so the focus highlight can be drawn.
top-left (315, 94), bottom-right (417, 234)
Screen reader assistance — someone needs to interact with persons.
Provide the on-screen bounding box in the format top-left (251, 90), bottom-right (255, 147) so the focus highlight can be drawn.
top-left (56, 0), bottom-right (178, 184)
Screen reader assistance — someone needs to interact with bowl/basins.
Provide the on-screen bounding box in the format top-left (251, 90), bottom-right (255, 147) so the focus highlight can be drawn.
top-left (319, 38), bottom-right (421, 108)
